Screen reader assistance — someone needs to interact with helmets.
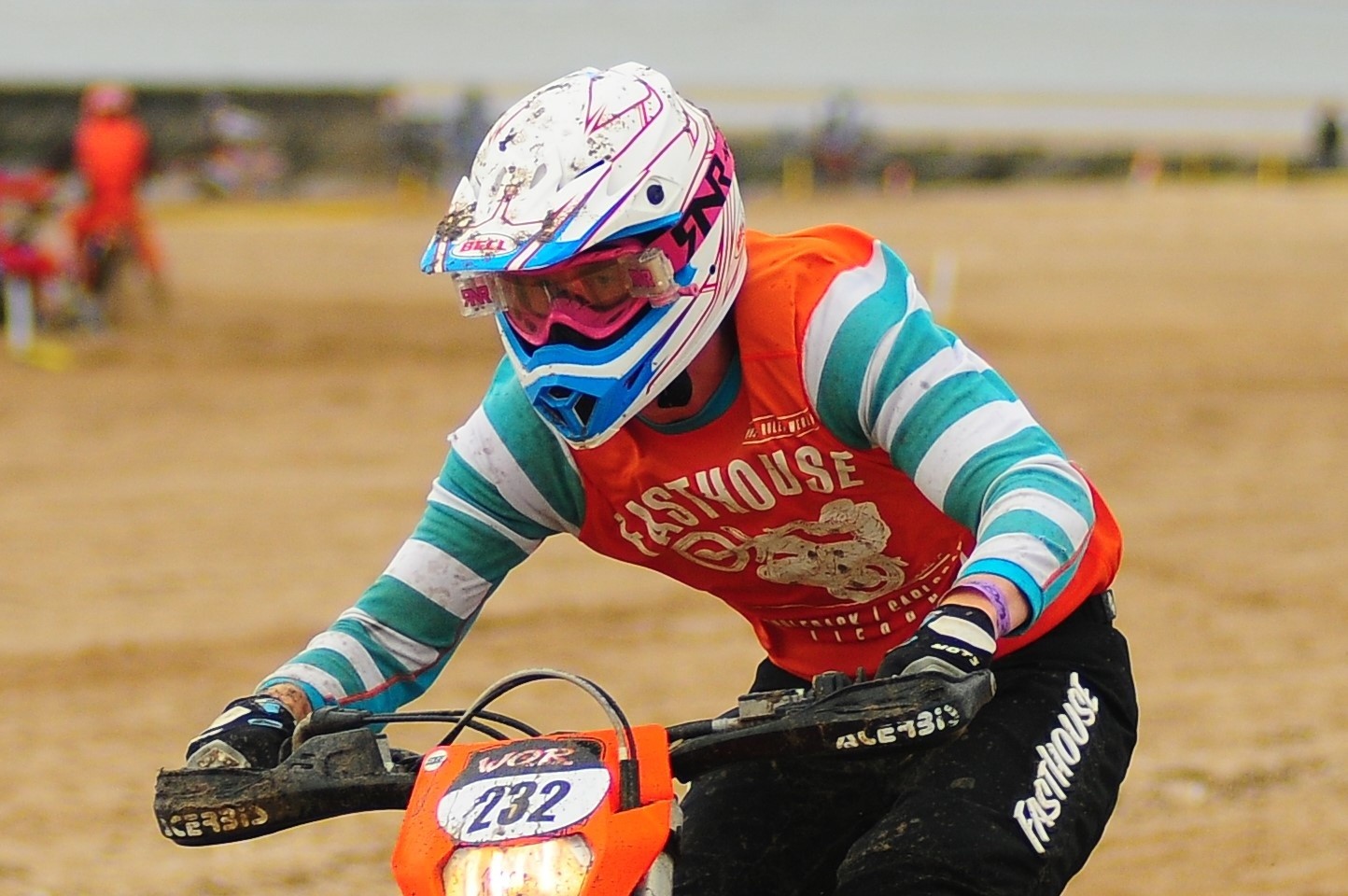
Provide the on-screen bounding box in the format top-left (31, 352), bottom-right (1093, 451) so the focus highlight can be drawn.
top-left (420, 61), bottom-right (748, 449)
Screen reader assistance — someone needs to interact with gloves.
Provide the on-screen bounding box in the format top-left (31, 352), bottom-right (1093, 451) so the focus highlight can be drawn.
top-left (187, 697), bottom-right (298, 769)
top-left (875, 605), bottom-right (996, 679)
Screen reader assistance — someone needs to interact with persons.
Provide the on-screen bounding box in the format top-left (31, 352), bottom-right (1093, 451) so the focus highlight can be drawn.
top-left (187, 62), bottom-right (1138, 896)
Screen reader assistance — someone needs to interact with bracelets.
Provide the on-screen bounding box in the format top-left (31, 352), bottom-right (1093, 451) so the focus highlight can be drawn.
top-left (939, 579), bottom-right (1011, 639)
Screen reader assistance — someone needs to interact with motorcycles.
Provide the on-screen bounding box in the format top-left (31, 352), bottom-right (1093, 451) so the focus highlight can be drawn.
top-left (148, 665), bottom-right (1025, 895)
